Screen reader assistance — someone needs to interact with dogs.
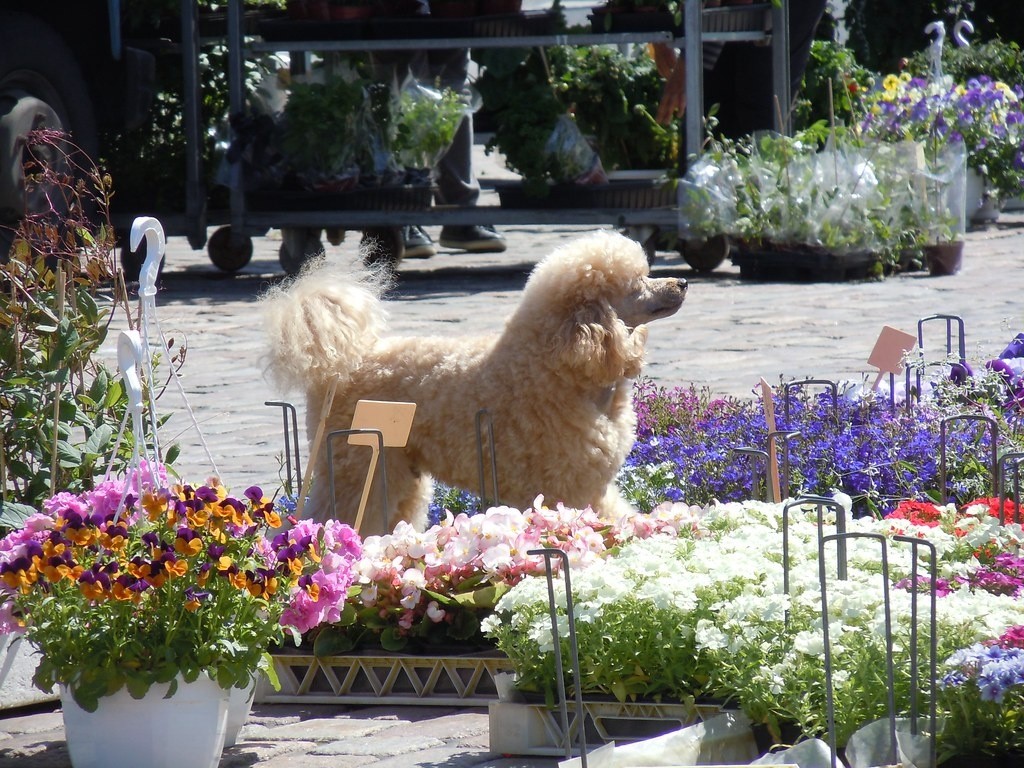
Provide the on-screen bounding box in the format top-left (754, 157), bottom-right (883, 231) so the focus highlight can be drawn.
top-left (262, 229), bottom-right (690, 545)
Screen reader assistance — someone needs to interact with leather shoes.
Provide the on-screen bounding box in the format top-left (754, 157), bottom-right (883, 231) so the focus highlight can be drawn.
top-left (439, 225), bottom-right (506, 252)
top-left (400, 226), bottom-right (436, 257)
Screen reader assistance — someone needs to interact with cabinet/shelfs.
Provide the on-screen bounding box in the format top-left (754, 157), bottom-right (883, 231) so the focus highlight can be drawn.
top-left (0, 2), bottom-right (226, 249)
top-left (228, 1), bottom-right (790, 224)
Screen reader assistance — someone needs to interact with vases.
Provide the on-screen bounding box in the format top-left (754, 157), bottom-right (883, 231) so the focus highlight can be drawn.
top-left (926, 241), bottom-right (964, 275)
top-left (975, 173), bottom-right (1000, 219)
top-left (740, 255), bottom-right (771, 280)
top-left (60, 668), bottom-right (258, 768)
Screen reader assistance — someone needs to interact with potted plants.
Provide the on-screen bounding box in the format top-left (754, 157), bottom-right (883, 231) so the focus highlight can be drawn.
top-left (0, 123), bottom-right (190, 710)
top-left (249, 50), bottom-right (469, 206)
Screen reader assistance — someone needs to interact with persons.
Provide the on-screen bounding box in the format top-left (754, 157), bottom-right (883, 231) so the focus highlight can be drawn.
top-left (649, 0), bottom-right (829, 263)
top-left (345, 0), bottom-right (506, 256)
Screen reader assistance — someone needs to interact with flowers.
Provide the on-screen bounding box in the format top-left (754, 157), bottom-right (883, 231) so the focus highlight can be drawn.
top-left (0, 460), bottom-right (366, 713)
top-left (672, 71), bottom-right (1024, 248)
top-left (0, 335), bottom-right (1024, 766)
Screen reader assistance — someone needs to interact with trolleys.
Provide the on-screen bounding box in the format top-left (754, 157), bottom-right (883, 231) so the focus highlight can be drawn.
top-left (1, 0), bottom-right (791, 278)
